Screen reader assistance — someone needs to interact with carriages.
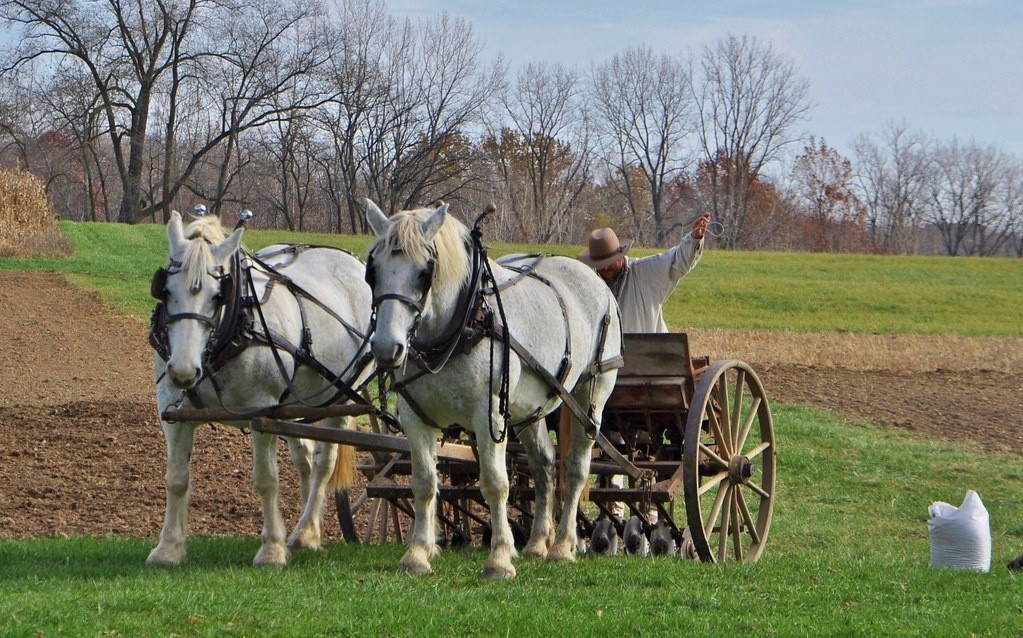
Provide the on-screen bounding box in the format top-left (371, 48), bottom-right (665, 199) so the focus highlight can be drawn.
top-left (145, 211), bottom-right (777, 586)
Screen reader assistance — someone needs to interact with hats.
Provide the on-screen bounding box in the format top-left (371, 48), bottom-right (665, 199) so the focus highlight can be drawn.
top-left (578, 227), bottom-right (634, 269)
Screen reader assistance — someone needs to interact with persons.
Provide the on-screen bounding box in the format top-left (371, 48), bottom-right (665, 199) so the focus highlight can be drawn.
top-left (575, 211), bottom-right (712, 524)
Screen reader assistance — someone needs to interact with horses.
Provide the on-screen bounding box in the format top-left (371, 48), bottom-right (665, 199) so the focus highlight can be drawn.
top-left (364, 198), bottom-right (621, 581)
top-left (145, 209), bottom-right (377, 567)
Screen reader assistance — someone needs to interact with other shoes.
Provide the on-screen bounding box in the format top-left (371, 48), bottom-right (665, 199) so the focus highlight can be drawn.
top-left (643, 512), bottom-right (657, 526)
top-left (600, 512), bottom-right (623, 525)
